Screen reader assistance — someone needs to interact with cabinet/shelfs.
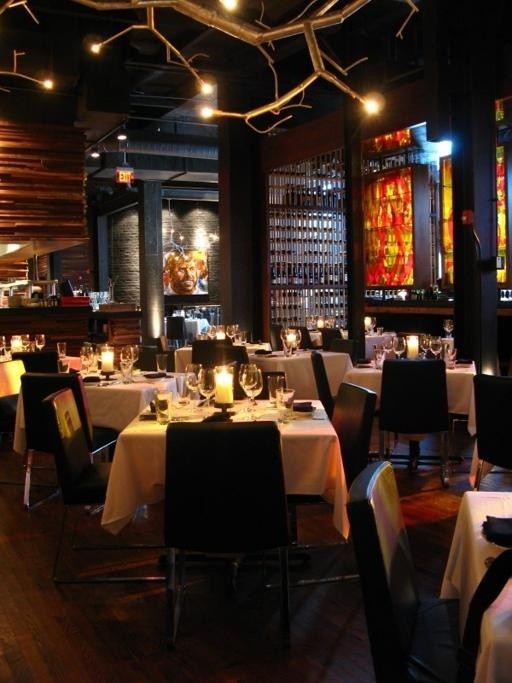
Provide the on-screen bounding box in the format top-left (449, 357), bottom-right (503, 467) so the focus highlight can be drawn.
top-left (96, 303), bottom-right (142, 352)
top-left (0, 305), bottom-right (94, 359)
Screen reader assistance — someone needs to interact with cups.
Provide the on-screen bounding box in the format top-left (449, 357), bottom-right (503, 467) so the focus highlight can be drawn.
top-left (183, 363), bottom-right (203, 405)
top-left (419, 336), bottom-right (432, 360)
top-left (20, 332), bottom-right (31, 351)
top-left (391, 336), bottom-right (407, 359)
top-left (34, 333), bottom-right (46, 352)
top-left (79, 343), bottom-right (94, 374)
top-left (56, 341), bottom-right (67, 357)
top-left (56, 357), bottom-right (71, 375)
top-left (266, 374), bottom-right (288, 408)
top-left (118, 344), bottom-right (140, 386)
top-left (239, 330), bottom-right (246, 346)
top-left (442, 319), bottom-right (455, 338)
top-left (207, 327), bottom-right (217, 340)
top-left (274, 388), bottom-right (297, 426)
top-left (238, 370), bottom-right (264, 412)
top-left (429, 335), bottom-right (443, 360)
top-left (154, 392), bottom-right (173, 427)
top-left (155, 353), bottom-right (169, 378)
top-left (196, 368), bottom-right (215, 416)
top-left (364, 316), bottom-right (377, 335)
top-left (280, 328), bottom-right (301, 356)
top-left (94, 342), bottom-right (110, 363)
top-left (225, 323), bottom-right (235, 345)
top-left (240, 362), bottom-right (260, 401)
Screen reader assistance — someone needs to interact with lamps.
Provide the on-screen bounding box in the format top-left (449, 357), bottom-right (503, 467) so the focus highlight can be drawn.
top-left (363, 316), bottom-right (373, 333)
top-left (212, 364), bottom-right (235, 407)
top-left (82, 0), bottom-right (419, 132)
top-left (0, 0), bottom-right (55, 92)
top-left (404, 335), bottom-right (421, 359)
top-left (316, 315), bottom-right (325, 329)
top-left (216, 324), bottom-right (227, 342)
top-left (100, 343), bottom-right (115, 371)
top-left (9, 335), bottom-right (23, 354)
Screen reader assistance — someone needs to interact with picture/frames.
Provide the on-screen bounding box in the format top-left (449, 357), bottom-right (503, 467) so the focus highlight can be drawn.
top-left (161, 250), bottom-right (210, 294)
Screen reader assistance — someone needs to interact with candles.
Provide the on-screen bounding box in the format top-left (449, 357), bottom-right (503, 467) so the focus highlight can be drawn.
top-left (287, 328), bottom-right (300, 349)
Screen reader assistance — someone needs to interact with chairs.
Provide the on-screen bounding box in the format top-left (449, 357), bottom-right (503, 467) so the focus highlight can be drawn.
top-left (471, 372), bottom-right (512, 493)
top-left (309, 350), bottom-right (337, 424)
top-left (286, 325), bottom-right (317, 352)
top-left (376, 357), bottom-right (452, 486)
top-left (348, 458), bottom-right (475, 683)
top-left (165, 316), bottom-right (186, 345)
top-left (138, 350), bottom-right (178, 375)
top-left (317, 325), bottom-right (344, 351)
top-left (17, 372), bottom-right (117, 512)
top-left (40, 386), bottom-right (178, 584)
top-left (161, 419), bottom-right (299, 652)
top-left (268, 324), bottom-right (285, 351)
top-left (326, 337), bottom-right (357, 365)
top-left (7, 350), bottom-right (62, 376)
top-left (174, 339), bottom-right (273, 402)
top-left (287, 382), bottom-right (377, 544)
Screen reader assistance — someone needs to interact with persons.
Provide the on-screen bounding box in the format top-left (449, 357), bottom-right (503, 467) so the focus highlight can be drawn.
top-left (165, 254), bottom-right (208, 295)
